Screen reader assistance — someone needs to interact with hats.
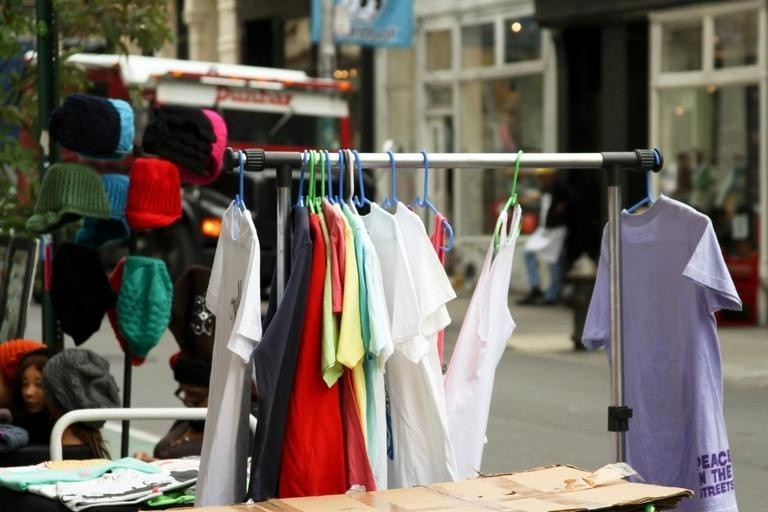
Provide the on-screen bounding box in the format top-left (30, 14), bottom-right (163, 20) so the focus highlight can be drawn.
top-left (104, 94), bottom-right (140, 156)
top-left (41, 346), bottom-right (123, 431)
top-left (18, 344), bottom-right (60, 366)
top-left (72, 170), bottom-right (133, 250)
top-left (166, 262), bottom-right (224, 352)
top-left (171, 347), bottom-right (213, 386)
top-left (105, 253), bottom-right (147, 368)
top-left (46, 236), bottom-right (118, 347)
top-left (181, 107), bottom-right (233, 190)
top-left (112, 253), bottom-right (175, 362)
top-left (140, 102), bottom-right (218, 179)
top-left (125, 153), bottom-right (186, 234)
top-left (45, 91), bottom-right (123, 160)
top-left (0, 335), bottom-right (48, 403)
top-left (0, 420), bottom-right (30, 455)
top-left (23, 161), bottom-right (113, 239)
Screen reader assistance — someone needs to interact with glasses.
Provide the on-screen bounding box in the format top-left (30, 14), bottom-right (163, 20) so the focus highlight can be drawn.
top-left (172, 386), bottom-right (210, 408)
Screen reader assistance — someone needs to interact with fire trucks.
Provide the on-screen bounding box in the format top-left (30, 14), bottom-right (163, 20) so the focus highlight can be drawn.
top-left (4, 40), bottom-right (366, 313)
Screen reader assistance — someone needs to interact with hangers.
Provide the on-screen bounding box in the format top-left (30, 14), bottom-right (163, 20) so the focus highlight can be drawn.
top-left (234, 149), bottom-right (524, 252)
top-left (627, 150), bottom-right (661, 214)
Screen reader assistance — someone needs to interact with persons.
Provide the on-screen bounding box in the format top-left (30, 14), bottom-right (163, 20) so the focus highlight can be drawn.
top-left (514, 167), bottom-right (572, 305)
top-left (0, 339), bottom-right (121, 460)
top-left (544, 169), bottom-right (608, 278)
top-left (132, 351), bottom-right (259, 463)
